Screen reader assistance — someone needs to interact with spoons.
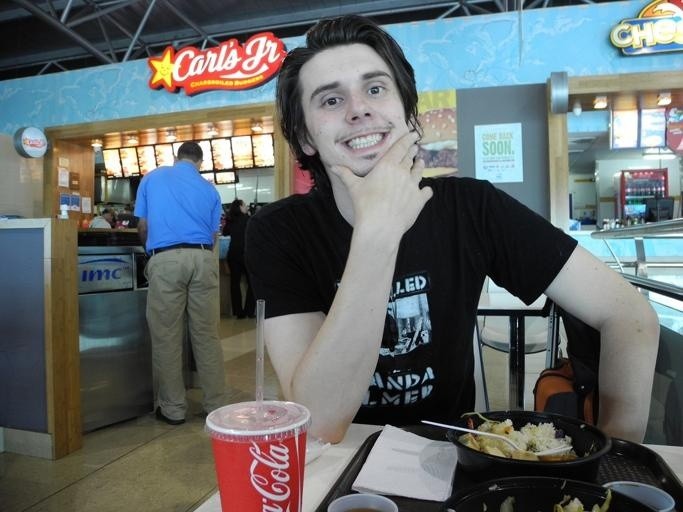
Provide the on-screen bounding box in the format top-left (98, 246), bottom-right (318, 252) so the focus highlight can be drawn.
top-left (421, 418), bottom-right (572, 456)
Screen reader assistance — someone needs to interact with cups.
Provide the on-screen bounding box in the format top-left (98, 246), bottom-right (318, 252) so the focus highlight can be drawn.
top-left (202, 399), bottom-right (312, 512)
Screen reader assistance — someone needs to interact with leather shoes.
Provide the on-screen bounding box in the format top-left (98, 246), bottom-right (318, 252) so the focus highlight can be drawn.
top-left (235, 312), bottom-right (257, 318)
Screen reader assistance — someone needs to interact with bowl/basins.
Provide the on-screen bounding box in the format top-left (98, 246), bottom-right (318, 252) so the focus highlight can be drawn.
top-left (445, 410), bottom-right (612, 483)
top-left (435, 475), bottom-right (659, 511)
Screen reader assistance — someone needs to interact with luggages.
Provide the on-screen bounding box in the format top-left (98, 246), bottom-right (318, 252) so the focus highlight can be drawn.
top-left (534, 304), bottom-right (599, 426)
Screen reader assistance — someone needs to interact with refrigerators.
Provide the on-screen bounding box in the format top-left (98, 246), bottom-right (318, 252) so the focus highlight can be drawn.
top-left (613, 168), bottom-right (670, 219)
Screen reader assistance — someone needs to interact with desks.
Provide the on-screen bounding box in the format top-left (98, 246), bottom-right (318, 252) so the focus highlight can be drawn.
top-left (194, 416), bottom-right (680, 512)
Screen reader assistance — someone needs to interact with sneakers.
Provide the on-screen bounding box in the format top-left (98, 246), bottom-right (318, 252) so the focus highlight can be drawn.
top-left (155, 406), bottom-right (186, 425)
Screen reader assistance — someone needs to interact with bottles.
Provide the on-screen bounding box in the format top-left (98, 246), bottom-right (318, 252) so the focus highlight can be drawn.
top-left (625, 180), bottom-right (664, 204)
top-left (601, 218), bottom-right (646, 229)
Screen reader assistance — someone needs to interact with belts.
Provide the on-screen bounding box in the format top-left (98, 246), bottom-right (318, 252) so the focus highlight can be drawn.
top-left (148, 242), bottom-right (213, 257)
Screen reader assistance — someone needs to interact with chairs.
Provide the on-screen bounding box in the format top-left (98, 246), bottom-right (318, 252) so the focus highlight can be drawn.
top-left (468, 296), bottom-right (567, 412)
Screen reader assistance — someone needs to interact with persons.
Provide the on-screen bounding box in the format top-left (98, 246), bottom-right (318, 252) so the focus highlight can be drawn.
top-left (219, 196), bottom-right (256, 318)
top-left (132, 141), bottom-right (227, 428)
top-left (238, 13), bottom-right (662, 451)
top-left (88, 206), bottom-right (115, 229)
top-left (117, 202), bottom-right (138, 229)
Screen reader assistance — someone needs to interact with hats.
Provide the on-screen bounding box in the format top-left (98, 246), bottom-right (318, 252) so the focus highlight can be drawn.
top-left (106, 208), bottom-right (116, 223)
